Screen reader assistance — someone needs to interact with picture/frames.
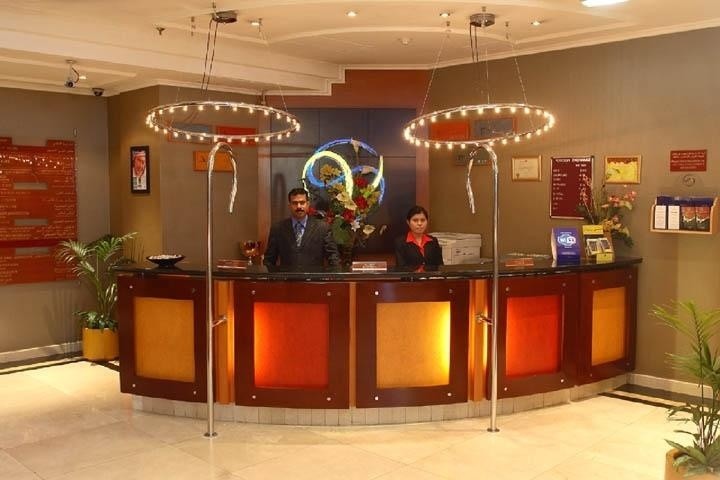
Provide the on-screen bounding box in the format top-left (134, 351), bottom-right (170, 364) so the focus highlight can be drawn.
top-left (130, 145), bottom-right (149, 193)
top-left (604, 155), bottom-right (642, 184)
top-left (511, 155), bottom-right (541, 182)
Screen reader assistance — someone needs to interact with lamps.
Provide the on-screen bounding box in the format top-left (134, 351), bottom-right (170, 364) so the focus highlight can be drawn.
top-left (401, 14), bottom-right (556, 151)
top-left (145, 18), bottom-right (301, 143)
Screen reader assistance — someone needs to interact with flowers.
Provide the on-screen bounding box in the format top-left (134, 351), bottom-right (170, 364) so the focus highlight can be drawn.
top-left (295, 165), bottom-right (387, 247)
top-left (579, 182), bottom-right (637, 248)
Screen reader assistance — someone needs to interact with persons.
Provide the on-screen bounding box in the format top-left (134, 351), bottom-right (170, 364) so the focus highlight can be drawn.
top-left (132, 151), bottom-right (146, 189)
top-left (394, 206), bottom-right (444, 271)
top-left (263, 189), bottom-right (341, 269)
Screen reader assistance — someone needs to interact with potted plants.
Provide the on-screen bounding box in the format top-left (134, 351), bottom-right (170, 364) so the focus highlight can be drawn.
top-left (54, 229), bottom-right (139, 363)
top-left (648, 297), bottom-right (720, 480)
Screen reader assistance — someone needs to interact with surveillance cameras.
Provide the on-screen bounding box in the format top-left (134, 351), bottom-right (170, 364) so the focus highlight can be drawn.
top-left (65, 80), bottom-right (76, 88)
top-left (93, 88), bottom-right (104, 97)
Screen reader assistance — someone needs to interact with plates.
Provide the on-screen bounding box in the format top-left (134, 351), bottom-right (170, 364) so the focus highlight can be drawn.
top-left (146, 255), bottom-right (186, 265)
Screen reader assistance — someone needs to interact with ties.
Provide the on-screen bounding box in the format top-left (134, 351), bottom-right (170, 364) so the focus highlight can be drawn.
top-left (294, 221), bottom-right (304, 247)
